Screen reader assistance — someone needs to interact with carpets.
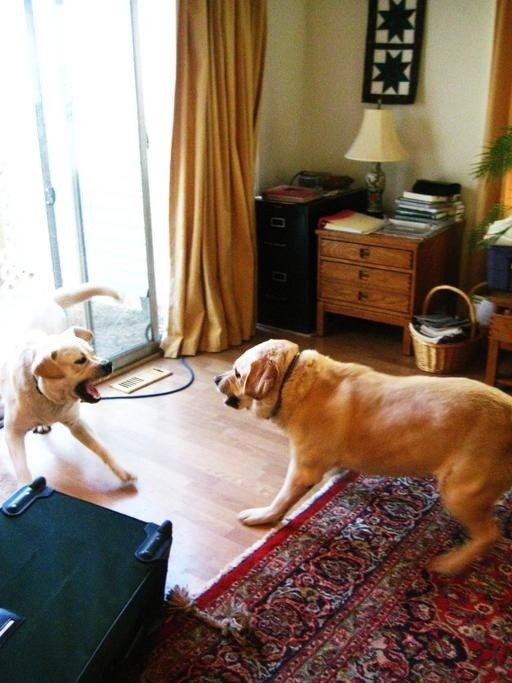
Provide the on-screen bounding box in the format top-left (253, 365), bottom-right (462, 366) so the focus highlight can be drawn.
top-left (147, 469), bottom-right (511, 683)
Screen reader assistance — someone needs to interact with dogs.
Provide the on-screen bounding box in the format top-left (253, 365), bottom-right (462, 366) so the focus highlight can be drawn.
top-left (213, 339), bottom-right (512, 578)
top-left (0, 285), bottom-right (139, 486)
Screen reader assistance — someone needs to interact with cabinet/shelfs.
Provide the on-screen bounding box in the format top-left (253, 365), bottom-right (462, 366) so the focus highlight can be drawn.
top-left (315, 214), bottom-right (462, 328)
top-left (256, 186), bottom-right (366, 335)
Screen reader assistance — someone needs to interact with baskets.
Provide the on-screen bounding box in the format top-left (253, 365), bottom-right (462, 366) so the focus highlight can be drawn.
top-left (408, 283), bottom-right (484, 376)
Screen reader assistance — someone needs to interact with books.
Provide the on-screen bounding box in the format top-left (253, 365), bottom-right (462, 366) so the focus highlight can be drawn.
top-left (317, 210), bottom-right (386, 234)
top-left (389, 190), bottom-right (465, 230)
top-left (408, 312), bottom-right (471, 344)
top-left (260, 184), bottom-right (322, 204)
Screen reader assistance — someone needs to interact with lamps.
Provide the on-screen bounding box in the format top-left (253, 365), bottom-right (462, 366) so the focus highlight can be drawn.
top-left (343, 100), bottom-right (410, 213)
